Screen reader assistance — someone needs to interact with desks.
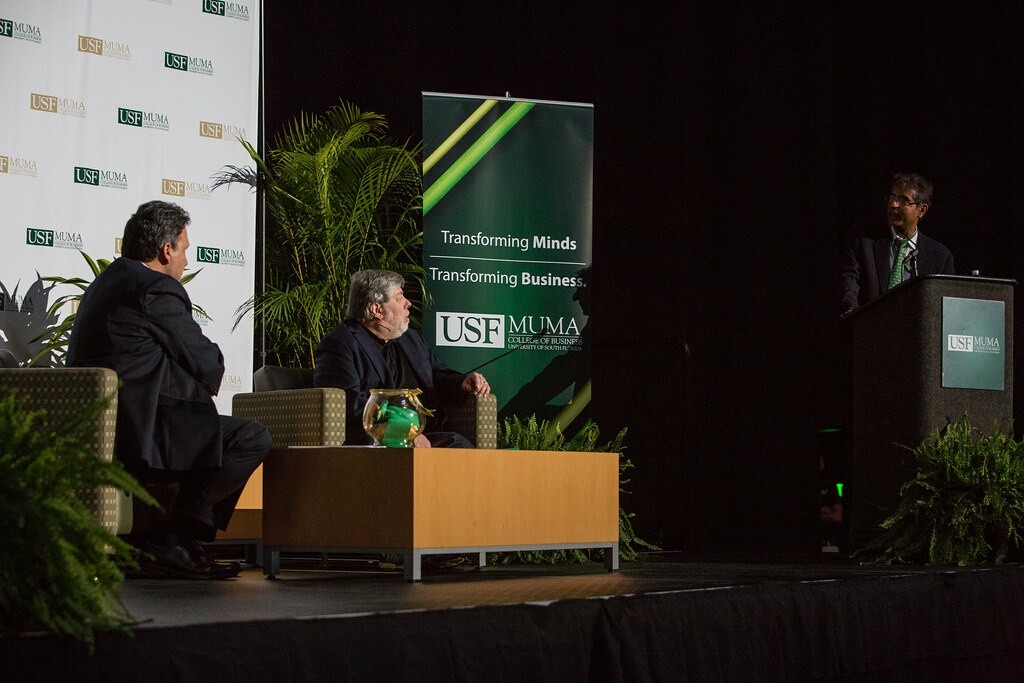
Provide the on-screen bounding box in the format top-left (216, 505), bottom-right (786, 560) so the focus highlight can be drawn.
top-left (261, 448), bottom-right (619, 581)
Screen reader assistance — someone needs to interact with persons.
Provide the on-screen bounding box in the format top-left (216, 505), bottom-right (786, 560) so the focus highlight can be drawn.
top-left (313, 269), bottom-right (491, 574)
top-left (836, 170), bottom-right (956, 318)
top-left (64, 199), bottom-right (272, 581)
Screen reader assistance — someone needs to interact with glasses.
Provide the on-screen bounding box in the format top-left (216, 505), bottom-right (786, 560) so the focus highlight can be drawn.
top-left (879, 191), bottom-right (921, 209)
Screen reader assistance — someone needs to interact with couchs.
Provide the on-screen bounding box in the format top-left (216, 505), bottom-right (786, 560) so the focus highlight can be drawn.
top-left (233, 368), bottom-right (498, 566)
top-left (0, 350), bottom-right (134, 554)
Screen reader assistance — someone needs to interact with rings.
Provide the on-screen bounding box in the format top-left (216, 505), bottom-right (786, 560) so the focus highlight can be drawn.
top-left (484, 382), bottom-right (487, 384)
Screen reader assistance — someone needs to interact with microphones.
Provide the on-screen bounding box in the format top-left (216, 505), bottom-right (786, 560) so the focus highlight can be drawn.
top-left (902, 249), bottom-right (919, 264)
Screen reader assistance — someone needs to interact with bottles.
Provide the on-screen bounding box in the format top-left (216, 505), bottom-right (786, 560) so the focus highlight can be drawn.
top-left (972, 269), bottom-right (980, 277)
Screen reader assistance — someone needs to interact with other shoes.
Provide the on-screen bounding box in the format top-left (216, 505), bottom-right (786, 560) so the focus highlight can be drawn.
top-left (820, 507), bottom-right (844, 555)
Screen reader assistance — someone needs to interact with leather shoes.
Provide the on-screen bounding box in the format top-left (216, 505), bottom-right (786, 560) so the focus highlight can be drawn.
top-left (125, 527), bottom-right (240, 584)
